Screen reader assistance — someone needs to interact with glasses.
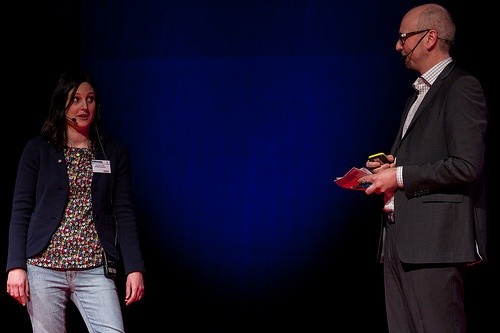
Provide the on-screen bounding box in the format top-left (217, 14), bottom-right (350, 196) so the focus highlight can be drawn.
top-left (399, 29), bottom-right (430, 43)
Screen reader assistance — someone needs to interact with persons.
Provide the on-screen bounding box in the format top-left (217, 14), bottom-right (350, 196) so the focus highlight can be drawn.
top-left (357, 3), bottom-right (491, 333)
top-left (6, 74), bottom-right (146, 332)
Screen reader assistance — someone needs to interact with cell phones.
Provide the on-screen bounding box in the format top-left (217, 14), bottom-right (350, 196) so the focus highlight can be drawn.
top-left (369, 153), bottom-right (391, 165)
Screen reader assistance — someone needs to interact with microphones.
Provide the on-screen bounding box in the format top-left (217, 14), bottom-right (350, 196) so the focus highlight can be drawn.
top-left (66, 115), bottom-right (76, 122)
top-left (402, 31), bottom-right (429, 61)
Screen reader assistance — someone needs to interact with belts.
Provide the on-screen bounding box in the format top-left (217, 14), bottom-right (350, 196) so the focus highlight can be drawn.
top-left (387, 213), bottom-right (395, 222)
